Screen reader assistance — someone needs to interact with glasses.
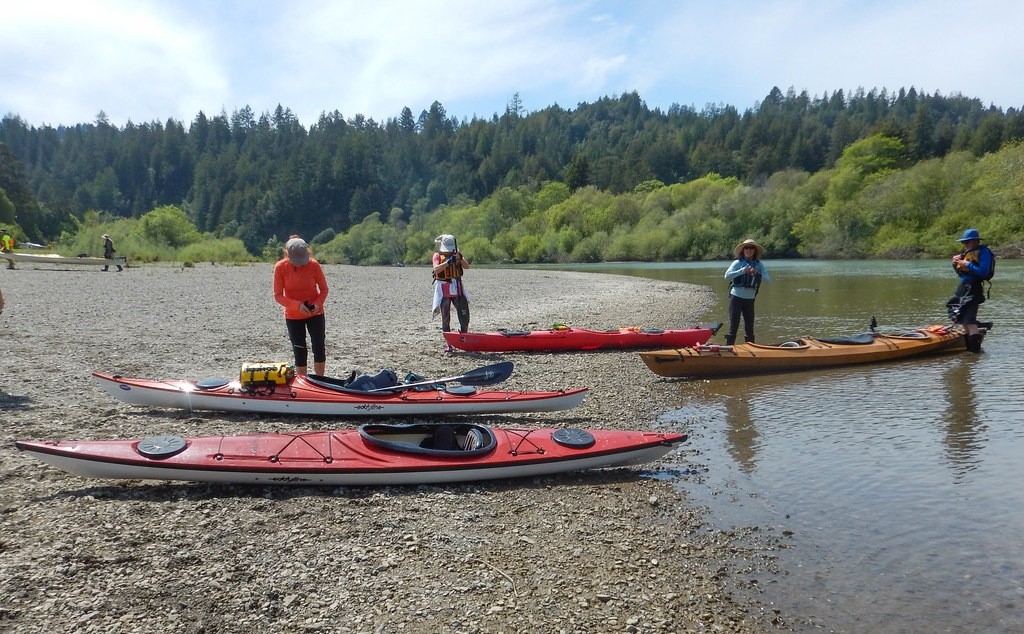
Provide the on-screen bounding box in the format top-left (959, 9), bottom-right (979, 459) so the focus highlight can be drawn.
top-left (744, 246), bottom-right (754, 249)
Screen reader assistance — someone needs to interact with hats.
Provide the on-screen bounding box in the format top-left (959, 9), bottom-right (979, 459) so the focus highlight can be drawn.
top-left (734, 239), bottom-right (764, 258)
top-left (102, 234), bottom-right (108, 239)
top-left (954, 229), bottom-right (984, 242)
top-left (439, 235), bottom-right (458, 253)
top-left (286, 238), bottom-right (310, 265)
top-left (0, 229), bottom-right (6, 232)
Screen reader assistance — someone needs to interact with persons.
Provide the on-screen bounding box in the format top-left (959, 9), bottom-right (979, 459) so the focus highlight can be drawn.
top-left (433, 235), bottom-right (470, 352)
top-left (102, 234), bottom-right (124, 272)
top-left (946, 229), bottom-right (995, 352)
top-left (1, 228), bottom-right (16, 269)
top-left (273, 234), bottom-right (329, 377)
top-left (724, 239), bottom-right (771, 350)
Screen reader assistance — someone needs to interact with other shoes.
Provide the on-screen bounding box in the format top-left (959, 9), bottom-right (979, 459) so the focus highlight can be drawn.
top-left (6, 268), bottom-right (14, 269)
top-left (116, 269), bottom-right (123, 272)
top-left (101, 269), bottom-right (108, 271)
top-left (445, 347), bottom-right (452, 352)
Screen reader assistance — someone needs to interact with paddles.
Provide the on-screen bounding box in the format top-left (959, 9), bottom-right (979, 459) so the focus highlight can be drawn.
top-left (369, 361), bottom-right (514, 391)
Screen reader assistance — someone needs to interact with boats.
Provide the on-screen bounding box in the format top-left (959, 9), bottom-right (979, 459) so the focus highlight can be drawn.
top-left (443, 322), bottom-right (725, 352)
top-left (637, 320), bottom-right (993, 379)
top-left (90, 360), bottom-right (590, 417)
top-left (13, 421), bottom-right (690, 489)
top-left (0, 251), bottom-right (126, 266)
